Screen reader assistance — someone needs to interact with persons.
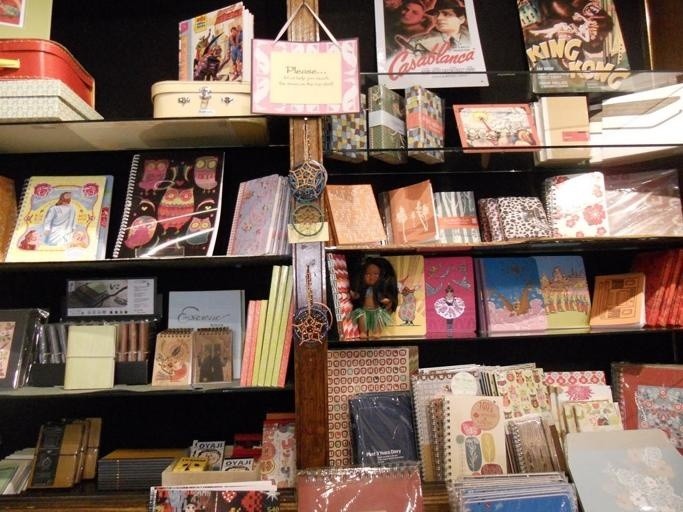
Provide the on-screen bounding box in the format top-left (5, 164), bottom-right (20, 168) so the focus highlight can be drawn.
top-left (525, 1), bottom-right (614, 70)
top-left (410, 0), bottom-right (472, 56)
top-left (384, 0), bottom-right (427, 58)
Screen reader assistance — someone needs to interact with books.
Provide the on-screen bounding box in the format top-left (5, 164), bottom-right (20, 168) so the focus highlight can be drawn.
top-left (173, 0), bottom-right (257, 84)
top-left (298, 346), bottom-right (683, 511)
top-left (327, 248), bottom-right (683, 340)
top-left (530, 96), bottom-right (592, 167)
top-left (326, 170), bottom-right (683, 246)
top-left (1, 413), bottom-right (295, 512)
top-left (0, 150), bottom-right (292, 262)
top-left (0, 266), bottom-right (294, 389)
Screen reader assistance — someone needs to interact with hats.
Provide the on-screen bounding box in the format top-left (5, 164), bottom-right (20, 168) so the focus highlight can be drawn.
top-left (425, 0), bottom-right (465, 17)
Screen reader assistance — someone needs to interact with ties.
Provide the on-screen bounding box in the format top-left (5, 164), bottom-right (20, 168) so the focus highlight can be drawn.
top-left (450, 37), bottom-right (455, 46)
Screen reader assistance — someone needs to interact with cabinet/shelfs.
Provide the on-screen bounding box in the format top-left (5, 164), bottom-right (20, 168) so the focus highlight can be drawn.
top-left (1, 0), bottom-right (683, 512)
